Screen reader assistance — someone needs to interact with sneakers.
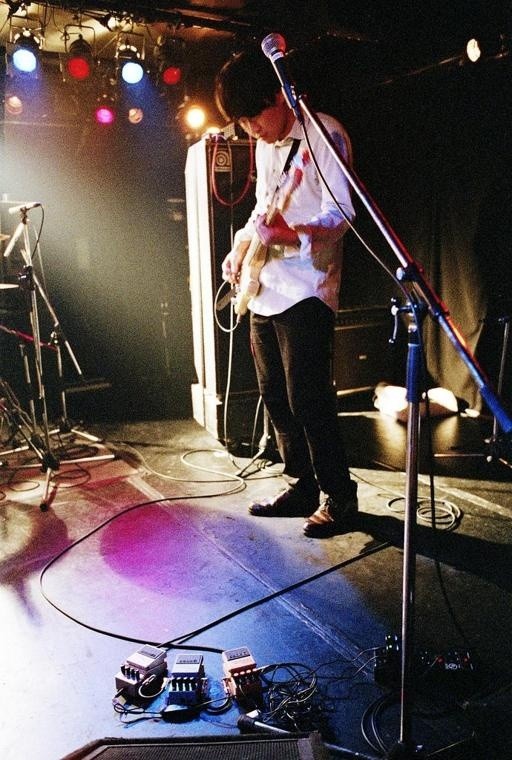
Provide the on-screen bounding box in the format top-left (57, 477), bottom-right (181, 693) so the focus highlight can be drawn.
top-left (303, 495), bottom-right (357, 539)
top-left (248, 486), bottom-right (321, 517)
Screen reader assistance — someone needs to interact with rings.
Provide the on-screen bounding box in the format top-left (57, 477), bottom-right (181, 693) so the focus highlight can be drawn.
top-left (260, 228), bottom-right (266, 235)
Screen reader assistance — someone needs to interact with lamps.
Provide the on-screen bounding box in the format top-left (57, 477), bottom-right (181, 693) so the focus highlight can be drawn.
top-left (2, 3), bottom-right (186, 125)
top-left (465, 33), bottom-right (509, 63)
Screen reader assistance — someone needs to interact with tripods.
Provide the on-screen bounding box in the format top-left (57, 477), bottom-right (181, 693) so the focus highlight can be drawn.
top-left (0, 345), bottom-right (46, 456)
top-left (238, 299), bottom-right (483, 760)
top-left (0, 211), bottom-right (122, 512)
top-left (18, 338), bottom-right (106, 444)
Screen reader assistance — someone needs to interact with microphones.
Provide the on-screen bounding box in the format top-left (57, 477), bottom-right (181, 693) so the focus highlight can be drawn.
top-left (261, 32), bottom-right (305, 126)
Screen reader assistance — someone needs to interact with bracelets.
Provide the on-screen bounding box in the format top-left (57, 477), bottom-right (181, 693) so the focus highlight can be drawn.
top-left (296, 236), bottom-right (302, 251)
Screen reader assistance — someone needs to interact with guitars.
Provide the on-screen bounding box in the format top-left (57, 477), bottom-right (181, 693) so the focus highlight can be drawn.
top-left (233, 149), bottom-right (311, 315)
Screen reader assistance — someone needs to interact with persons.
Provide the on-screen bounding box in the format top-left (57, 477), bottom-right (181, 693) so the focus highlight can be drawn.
top-left (215, 50), bottom-right (360, 538)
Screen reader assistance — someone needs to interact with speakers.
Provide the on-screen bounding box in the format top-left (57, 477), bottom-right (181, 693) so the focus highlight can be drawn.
top-left (61, 731), bottom-right (328, 760)
